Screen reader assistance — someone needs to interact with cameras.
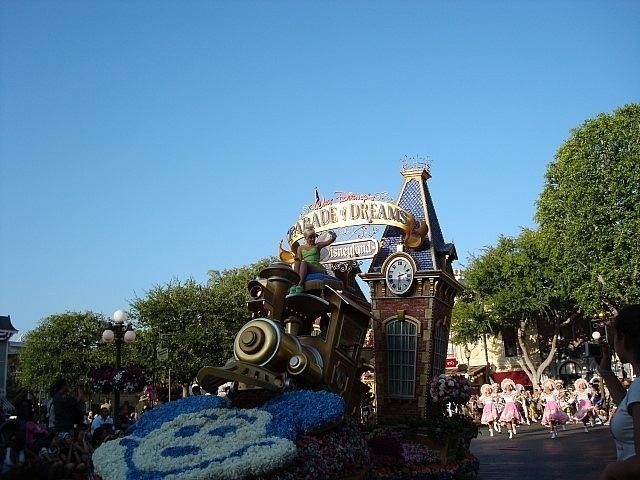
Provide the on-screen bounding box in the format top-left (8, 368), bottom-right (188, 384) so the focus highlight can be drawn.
top-left (585, 343), bottom-right (603, 358)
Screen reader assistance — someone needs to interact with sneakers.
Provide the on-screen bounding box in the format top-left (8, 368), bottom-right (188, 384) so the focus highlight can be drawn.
top-left (583, 418), bottom-right (609, 432)
top-left (526, 414), bottom-right (541, 426)
top-left (489, 423), bottom-right (502, 437)
top-left (542, 422), bottom-right (566, 439)
top-left (509, 428), bottom-right (518, 439)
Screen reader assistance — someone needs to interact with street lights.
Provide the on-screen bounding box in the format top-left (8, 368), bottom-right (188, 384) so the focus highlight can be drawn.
top-left (592, 331), bottom-right (608, 410)
top-left (102, 310), bottom-right (136, 430)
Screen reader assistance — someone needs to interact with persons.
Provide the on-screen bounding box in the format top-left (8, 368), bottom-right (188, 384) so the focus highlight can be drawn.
top-left (598, 304), bottom-right (640, 480)
top-left (458, 372), bottom-right (632, 441)
top-left (0, 373), bottom-right (136, 480)
top-left (288, 224), bottom-right (337, 293)
top-left (192, 386), bottom-right (201, 395)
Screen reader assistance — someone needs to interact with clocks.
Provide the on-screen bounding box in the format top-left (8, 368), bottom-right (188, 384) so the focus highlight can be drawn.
top-left (385, 257), bottom-right (414, 296)
